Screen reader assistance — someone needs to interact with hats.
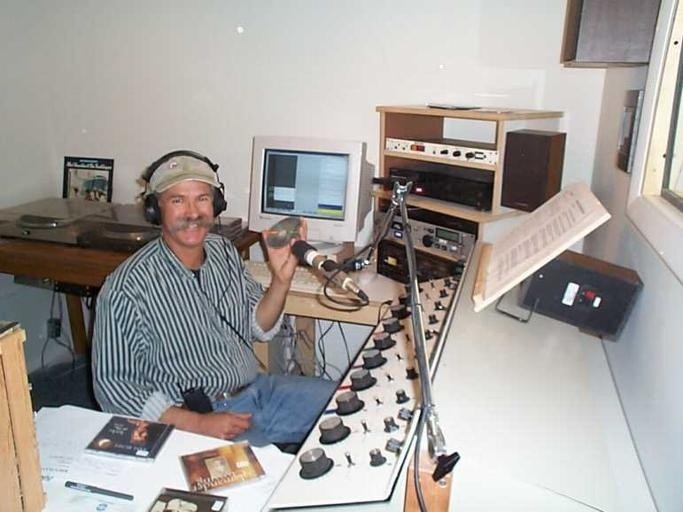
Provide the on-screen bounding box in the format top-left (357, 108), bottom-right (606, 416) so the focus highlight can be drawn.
top-left (143, 151), bottom-right (221, 200)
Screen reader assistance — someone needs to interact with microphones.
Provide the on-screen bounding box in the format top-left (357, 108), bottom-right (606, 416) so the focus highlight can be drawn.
top-left (290, 239), bottom-right (369, 302)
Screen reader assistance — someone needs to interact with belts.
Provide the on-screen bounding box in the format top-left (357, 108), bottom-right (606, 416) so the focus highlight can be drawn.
top-left (219, 383), bottom-right (250, 399)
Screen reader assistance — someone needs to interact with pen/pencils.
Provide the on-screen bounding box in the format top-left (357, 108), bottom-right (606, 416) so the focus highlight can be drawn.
top-left (65, 482), bottom-right (134, 503)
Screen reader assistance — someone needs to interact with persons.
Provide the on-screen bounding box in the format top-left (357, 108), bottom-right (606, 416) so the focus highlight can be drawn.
top-left (89, 151), bottom-right (340, 448)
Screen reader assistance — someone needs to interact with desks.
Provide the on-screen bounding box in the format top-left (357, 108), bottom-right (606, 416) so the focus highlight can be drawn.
top-left (241, 258), bottom-right (385, 377)
top-left (2, 232), bottom-right (261, 365)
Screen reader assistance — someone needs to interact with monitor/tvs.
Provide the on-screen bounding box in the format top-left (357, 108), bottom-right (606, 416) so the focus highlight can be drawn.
top-left (247, 134), bottom-right (375, 254)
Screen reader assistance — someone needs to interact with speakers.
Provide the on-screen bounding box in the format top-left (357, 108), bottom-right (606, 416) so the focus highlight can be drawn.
top-left (518, 248), bottom-right (646, 342)
top-left (501, 126), bottom-right (568, 213)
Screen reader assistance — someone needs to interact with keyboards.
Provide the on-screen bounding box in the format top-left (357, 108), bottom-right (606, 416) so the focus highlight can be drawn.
top-left (243, 260), bottom-right (363, 296)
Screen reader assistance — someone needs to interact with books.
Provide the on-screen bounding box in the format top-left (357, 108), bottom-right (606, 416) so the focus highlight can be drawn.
top-left (85, 415), bottom-right (175, 461)
top-left (149, 488), bottom-right (228, 512)
top-left (180, 439), bottom-right (266, 493)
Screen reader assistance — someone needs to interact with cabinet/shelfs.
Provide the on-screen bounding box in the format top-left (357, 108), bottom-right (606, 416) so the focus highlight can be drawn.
top-left (372, 105), bottom-right (563, 263)
